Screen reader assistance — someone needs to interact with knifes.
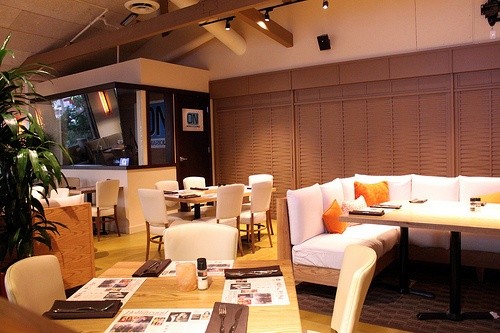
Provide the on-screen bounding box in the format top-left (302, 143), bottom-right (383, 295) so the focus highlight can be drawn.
top-left (229, 308), bottom-right (243, 333)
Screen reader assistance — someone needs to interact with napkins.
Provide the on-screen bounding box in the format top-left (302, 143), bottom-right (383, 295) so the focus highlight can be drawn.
top-left (44, 258), bottom-right (171, 319)
top-left (206, 265), bottom-right (282, 332)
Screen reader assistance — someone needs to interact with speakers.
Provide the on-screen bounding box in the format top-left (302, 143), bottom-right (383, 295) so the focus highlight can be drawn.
top-left (316, 34), bottom-right (330, 51)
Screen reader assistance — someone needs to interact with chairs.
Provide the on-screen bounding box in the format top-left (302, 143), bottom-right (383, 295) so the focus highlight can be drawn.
top-left (30, 176), bottom-right (120, 241)
top-left (184, 176), bottom-right (212, 213)
top-left (137, 189), bottom-right (174, 261)
top-left (192, 182), bottom-right (244, 256)
top-left (154, 179), bottom-right (190, 220)
top-left (242, 174), bottom-right (274, 233)
top-left (164, 224), bottom-right (239, 260)
top-left (308, 245), bottom-right (379, 333)
top-left (5, 255), bottom-right (66, 316)
top-left (239, 179), bottom-right (274, 253)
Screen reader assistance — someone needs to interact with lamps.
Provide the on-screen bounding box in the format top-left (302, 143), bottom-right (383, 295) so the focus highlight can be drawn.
top-left (225, 0), bottom-right (330, 31)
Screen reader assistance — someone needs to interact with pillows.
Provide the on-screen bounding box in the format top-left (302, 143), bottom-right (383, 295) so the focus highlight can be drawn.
top-left (472, 192), bottom-right (500, 204)
top-left (323, 199), bottom-right (346, 235)
top-left (354, 181), bottom-right (389, 205)
top-left (343, 195), bottom-right (368, 225)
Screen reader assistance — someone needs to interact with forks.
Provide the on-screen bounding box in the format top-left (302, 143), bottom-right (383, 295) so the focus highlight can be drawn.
top-left (219, 303), bottom-right (226, 333)
top-left (55, 303), bottom-right (114, 311)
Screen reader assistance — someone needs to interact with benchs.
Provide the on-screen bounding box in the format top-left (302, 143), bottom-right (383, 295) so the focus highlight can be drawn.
top-left (276, 173), bottom-right (500, 288)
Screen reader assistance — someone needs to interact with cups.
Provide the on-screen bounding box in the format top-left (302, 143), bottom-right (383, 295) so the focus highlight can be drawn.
top-left (175, 262), bottom-right (197, 292)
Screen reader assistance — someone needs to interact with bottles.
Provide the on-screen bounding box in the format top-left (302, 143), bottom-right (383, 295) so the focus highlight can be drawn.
top-left (470, 198), bottom-right (476, 212)
top-left (476, 198), bottom-right (481, 207)
top-left (196, 258), bottom-right (208, 290)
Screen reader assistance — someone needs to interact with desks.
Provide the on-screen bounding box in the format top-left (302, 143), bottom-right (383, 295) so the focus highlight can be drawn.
top-left (43, 258), bottom-right (301, 333)
top-left (341, 200), bottom-right (500, 321)
top-left (165, 185), bottom-right (276, 220)
